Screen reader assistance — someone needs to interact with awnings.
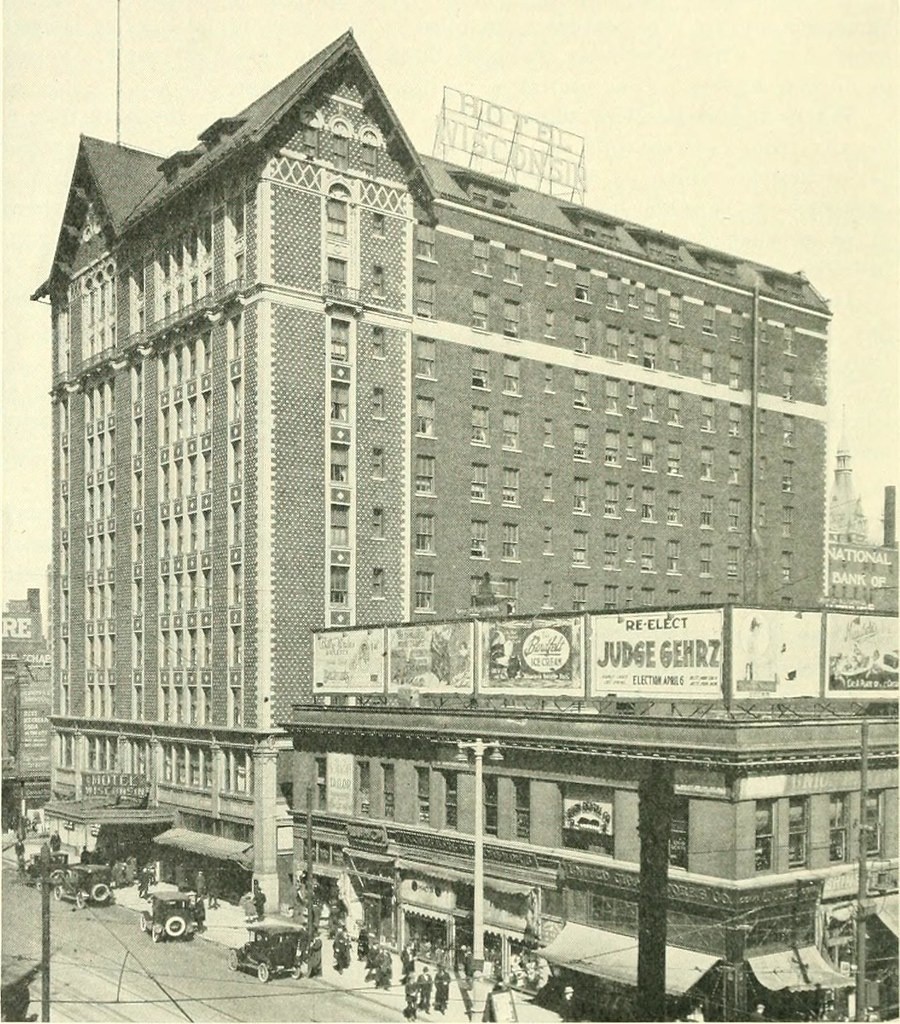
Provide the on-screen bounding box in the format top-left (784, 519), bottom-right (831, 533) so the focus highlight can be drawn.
top-left (154, 827), bottom-right (254, 860)
top-left (870, 891), bottom-right (898, 942)
top-left (747, 944), bottom-right (858, 994)
top-left (529, 921), bottom-right (724, 999)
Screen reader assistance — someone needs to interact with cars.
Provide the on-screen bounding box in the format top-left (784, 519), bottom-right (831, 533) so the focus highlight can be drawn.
top-left (24, 850), bottom-right (68, 892)
top-left (226, 920), bottom-right (306, 983)
top-left (138, 889), bottom-right (196, 943)
top-left (51, 863), bottom-right (113, 911)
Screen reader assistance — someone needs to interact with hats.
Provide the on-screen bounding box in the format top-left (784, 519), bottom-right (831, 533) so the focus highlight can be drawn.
top-left (423, 967), bottom-right (429, 973)
top-left (564, 986), bottom-right (574, 993)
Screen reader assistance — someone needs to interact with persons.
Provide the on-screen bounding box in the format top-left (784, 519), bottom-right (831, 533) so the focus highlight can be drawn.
top-left (299, 885), bottom-right (552, 1021)
top-left (686, 1003), bottom-right (705, 1021)
top-left (174, 861), bottom-right (221, 934)
top-left (559, 985), bottom-right (578, 1021)
top-left (8, 811), bottom-right (62, 871)
top-left (749, 1003), bottom-right (766, 1021)
top-left (243, 888), bottom-right (266, 923)
top-left (81, 845), bottom-right (157, 899)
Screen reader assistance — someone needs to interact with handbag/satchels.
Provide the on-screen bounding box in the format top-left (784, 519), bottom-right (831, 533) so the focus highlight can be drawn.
top-left (334, 952), bottom-right (341, 960)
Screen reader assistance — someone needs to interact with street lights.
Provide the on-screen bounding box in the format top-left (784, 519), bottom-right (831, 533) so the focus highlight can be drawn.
top-left (454, 736), bottom-right (505, 1022)
